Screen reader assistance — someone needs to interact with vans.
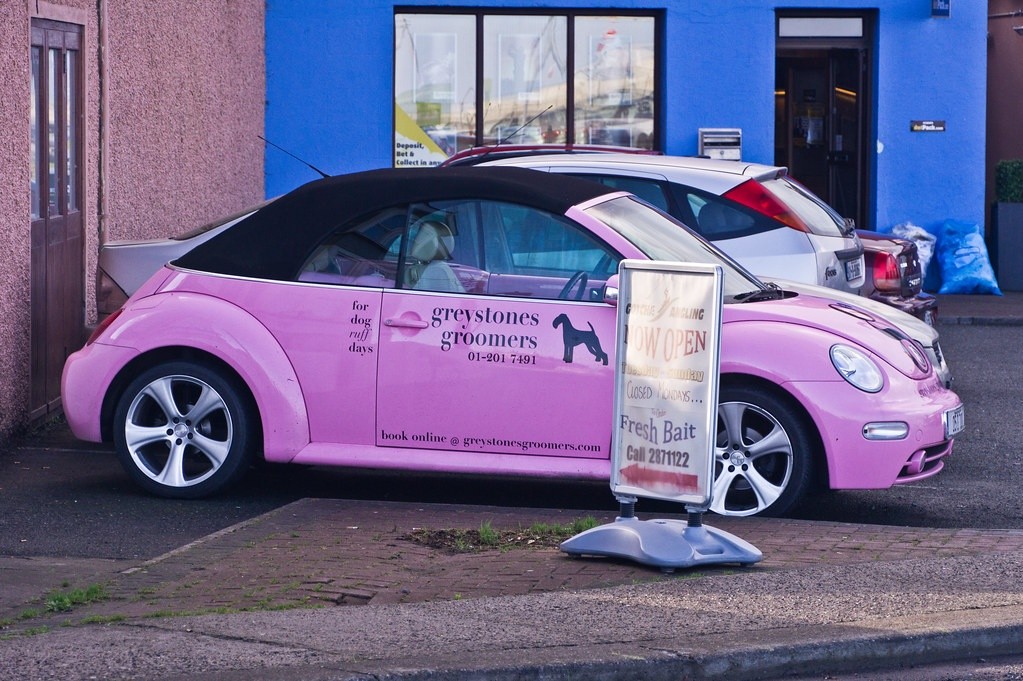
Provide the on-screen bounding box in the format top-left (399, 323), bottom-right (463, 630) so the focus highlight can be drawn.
top-left (423, 111), bottom-right (654, 151)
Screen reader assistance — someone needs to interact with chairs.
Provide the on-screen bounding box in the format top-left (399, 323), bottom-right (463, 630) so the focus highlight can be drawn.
top-left (402, 219), bottom-right (467, 293)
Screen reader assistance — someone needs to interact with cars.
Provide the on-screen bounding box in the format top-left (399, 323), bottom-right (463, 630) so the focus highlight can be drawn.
top-left (62, 166), bottom-right (968, 523)
top-left (423, 144), bottom-right (940, 327)
top-left (385, 153), bottom-right (869, 303)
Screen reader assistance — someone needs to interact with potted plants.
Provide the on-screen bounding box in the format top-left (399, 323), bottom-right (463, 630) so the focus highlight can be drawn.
top-left (991, 159), bottom-right (1023, 291)
